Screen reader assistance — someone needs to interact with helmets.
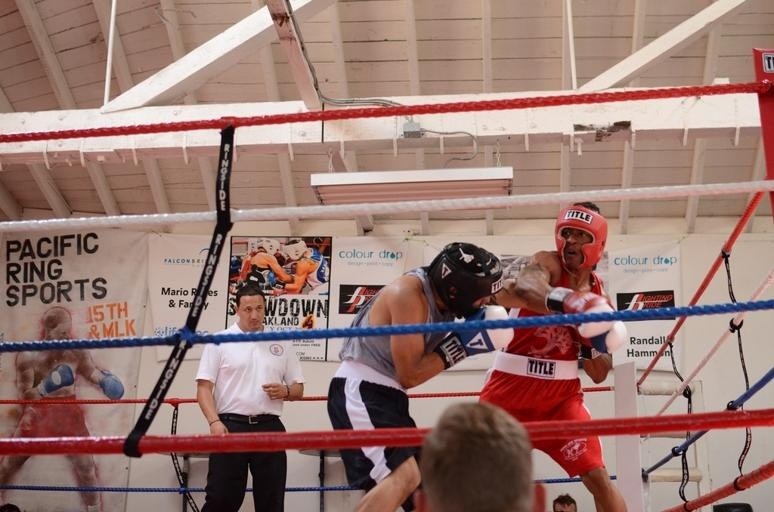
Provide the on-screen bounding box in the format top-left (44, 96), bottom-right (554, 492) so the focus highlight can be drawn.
top-left (553, 202), bottom-right (610, 276)
top-left (426, 240), bottom-right (505, 318)
top-left (258, 237), bottom-right (281, 255)
top-left (284, 239), bottom-right (310, 262)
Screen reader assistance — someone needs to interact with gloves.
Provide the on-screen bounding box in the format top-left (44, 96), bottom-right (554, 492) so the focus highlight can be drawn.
top-left (37, 364), bottom-right (76, 397)
top-left (550, 288), bottom-right (616, 361)
top-left (431, 305), bottom-right (515, 374)
top-left (261, 270), bottom-right (277, 286)
top-left (91, 366), bottom-right (124, 399)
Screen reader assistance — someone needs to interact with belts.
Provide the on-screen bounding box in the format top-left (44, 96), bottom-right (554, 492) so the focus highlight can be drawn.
top-left (211, 411), bottom-right (278, 425)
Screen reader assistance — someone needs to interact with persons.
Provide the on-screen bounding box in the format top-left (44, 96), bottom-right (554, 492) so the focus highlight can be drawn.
top-left (419, 401), bottom-right (547, 512)
top-left (195, 285), bottom-right (305, 512)
top-left (327, 242), bottom-right (627, 512)
top-left (479, 202), bottom-right (627, 512)
top-left (0, 306), bottom-right (124, 512)
top-left (553, 494), bottom-right (577, 512)
top-left (236, 239), bottom-right (295, 290)
top-left (271, 239), bottom-right (330, 295)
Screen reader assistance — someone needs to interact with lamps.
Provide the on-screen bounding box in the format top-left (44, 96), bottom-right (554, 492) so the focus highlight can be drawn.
top-left (310, 167), bottom-right (514, 206)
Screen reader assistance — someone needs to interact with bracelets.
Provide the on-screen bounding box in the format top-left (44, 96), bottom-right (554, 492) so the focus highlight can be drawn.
top-left (209, 419), bottom-right (221, 426)
top-left (284, 385), bottom-right (290, 399)
